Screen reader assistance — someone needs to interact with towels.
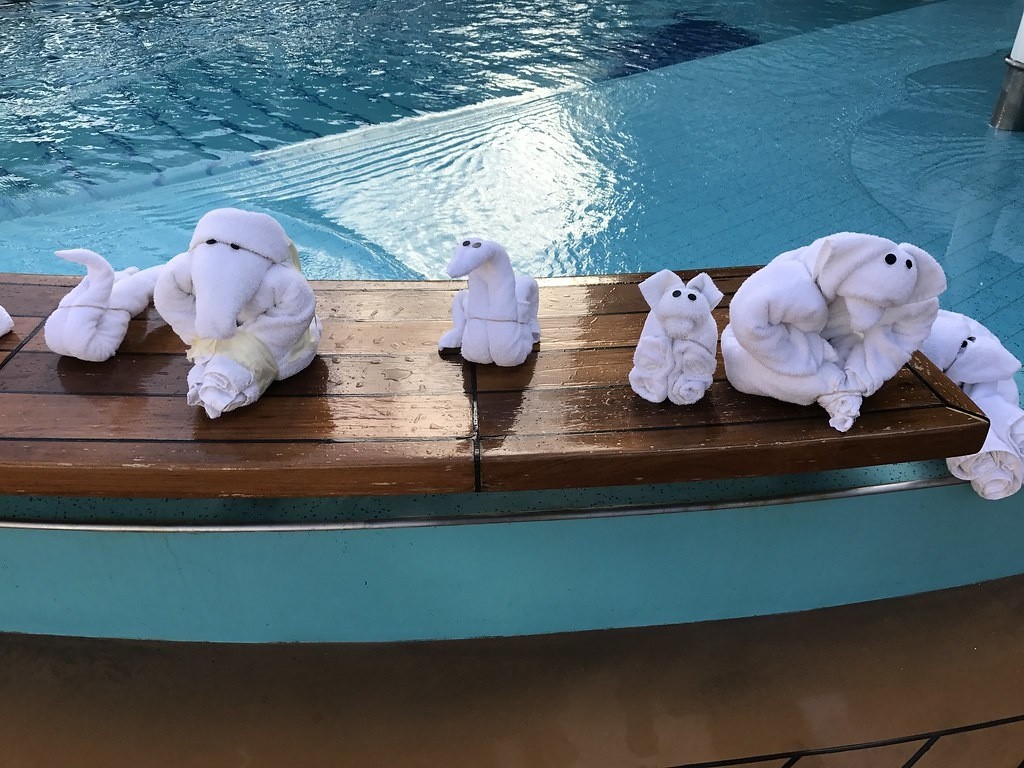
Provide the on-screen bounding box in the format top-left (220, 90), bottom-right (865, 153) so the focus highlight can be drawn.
top-left (437, 238), bottom-right (542, 369)
top-left (41, 246), bottom-right (165, 361)
top-left (627, 268), bottom-right (724, 405)
top-left (916, 308), bottom-right (1023, 501)
top-left (0, 302), bottom-right (15, 337)
top-left (722, 230), bottom-right (951, 432)
top-left (152, 208), bottom-right (322, 420)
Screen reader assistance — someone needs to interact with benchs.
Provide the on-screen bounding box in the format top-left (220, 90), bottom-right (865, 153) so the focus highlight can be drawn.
top-left (0, 267), bottom-right (991, 647)
